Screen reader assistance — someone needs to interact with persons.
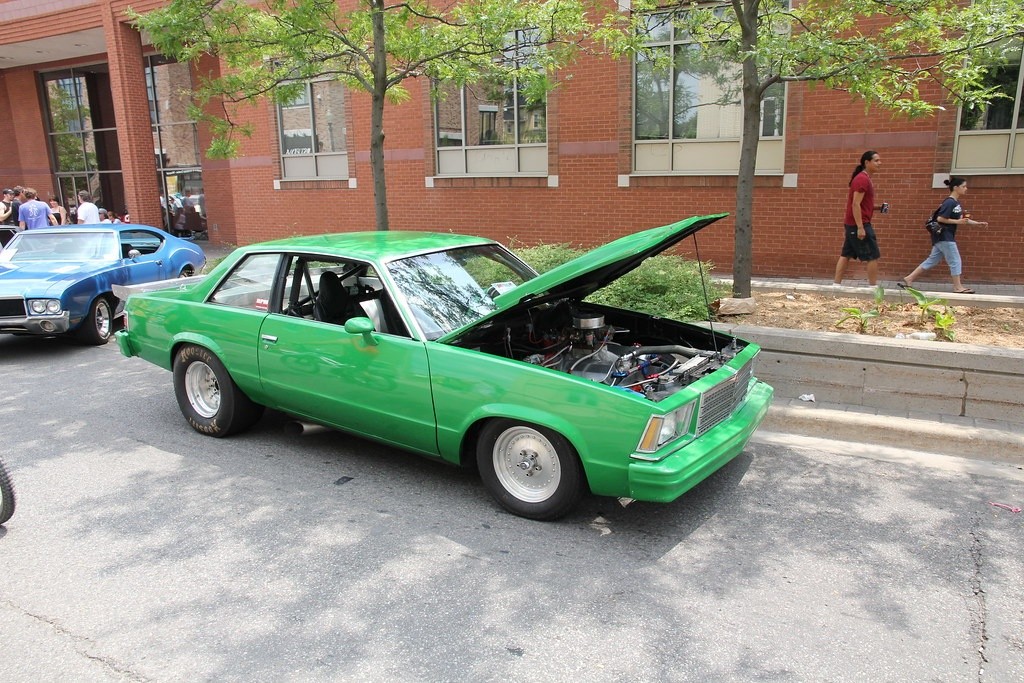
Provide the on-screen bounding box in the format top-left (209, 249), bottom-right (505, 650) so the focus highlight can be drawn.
top-left (11, 186), bottom-right (27, 231)
top-left (76, 189), bottom-right (101, 224)
top-left (108, 210), bottom-right (122, 224)
top-left (832, 151), bottom-right (891, 288)
top-left (45, 193), bottom-right (67, 225)
top-left (18, 187), bottom-right (59, 233)
top-left (156, 187), bottom-right (207, 237)
top-left (96, 207), bottom-right (112, 224)
top-left (0, 186), bottom-right (14, 225)
top-left (902, 177), bottom-right (988, 293)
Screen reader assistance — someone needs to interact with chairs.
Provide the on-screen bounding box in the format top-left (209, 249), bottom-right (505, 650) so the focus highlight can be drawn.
top-left (316, 271), bottom-right (377, 332)
top-left (121, 244), bottom-right (133, 258)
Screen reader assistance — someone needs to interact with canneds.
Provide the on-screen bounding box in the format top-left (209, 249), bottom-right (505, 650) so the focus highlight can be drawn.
top-left (881, 203), bottom-right (889, 214)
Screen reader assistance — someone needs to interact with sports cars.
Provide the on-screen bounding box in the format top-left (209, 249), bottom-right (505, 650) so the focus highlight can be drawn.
top-left (0, 224), bottom-right (207, 347)
top-left (120, 209), bottom-right (775, 521)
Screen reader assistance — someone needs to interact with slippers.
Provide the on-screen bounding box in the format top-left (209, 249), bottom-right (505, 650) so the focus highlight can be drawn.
top-left (897, 283), bottom-right (912, 290)
top-left (953, 289), bottom-right (975, 294)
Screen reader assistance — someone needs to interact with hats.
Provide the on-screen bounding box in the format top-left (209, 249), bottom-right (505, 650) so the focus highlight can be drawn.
top-left (3, 188), bottom-right (14, 194)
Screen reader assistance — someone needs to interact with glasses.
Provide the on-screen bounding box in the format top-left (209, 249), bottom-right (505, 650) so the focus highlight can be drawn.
top-left (99, 212), bottom-right (103, 214)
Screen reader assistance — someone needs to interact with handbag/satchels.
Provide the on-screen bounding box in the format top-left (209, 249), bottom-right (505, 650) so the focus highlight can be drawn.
top-left (925, 197), bottom-right (957, 236)
